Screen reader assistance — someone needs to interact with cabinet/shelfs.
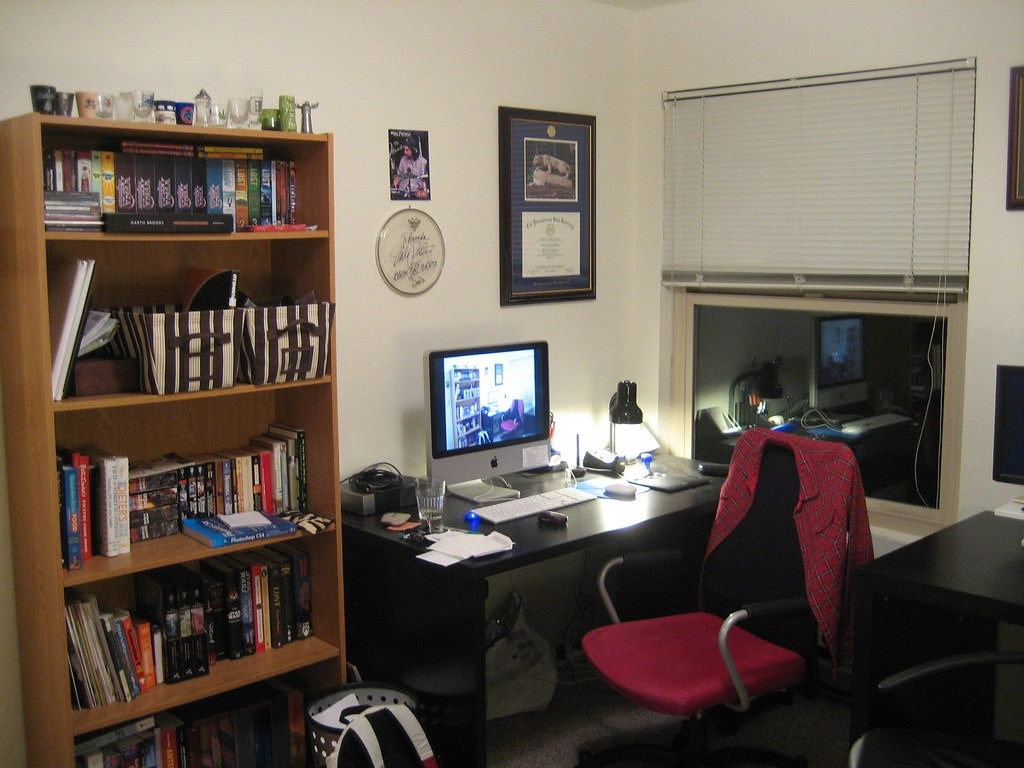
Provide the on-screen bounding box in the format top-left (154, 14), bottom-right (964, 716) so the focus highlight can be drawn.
top-left (0, 111), bottom-right (347, 768)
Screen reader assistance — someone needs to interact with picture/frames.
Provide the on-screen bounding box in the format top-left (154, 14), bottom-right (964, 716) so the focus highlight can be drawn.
top-left (1006, 65), bottom-right (1024, 210)
top-left (498, 106), bottom-right (598, 306)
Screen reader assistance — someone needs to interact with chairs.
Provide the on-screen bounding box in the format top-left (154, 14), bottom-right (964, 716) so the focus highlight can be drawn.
top-left (849, 656), bottom-right (1024, 767)
top-left (582, 427), bottom-right (874, 767)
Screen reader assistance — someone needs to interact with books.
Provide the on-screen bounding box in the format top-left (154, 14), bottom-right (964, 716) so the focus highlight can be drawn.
top-left (44, 142), bottom-right (319, 233)
top-left (56, 424), bottom-right (307, 571)
top-left (64, 542), bottom-right (312, 711)
top-left (74, 676), bottom-right (308, 768)
top-left (46, 257), bottom-right (119, 402)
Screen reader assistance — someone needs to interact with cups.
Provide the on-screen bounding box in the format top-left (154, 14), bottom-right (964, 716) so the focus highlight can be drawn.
top-left (415, 475), bottom-right (445, 520)
top-left (55, 92), bottom-right (75, 117)
top-left (228, 99), bottom-right (249, 130)
top-left (261, 109), bottom-right (281, 131)
top-left (154, 100), bottom-right (177, 124)
top-left (176, 102), bottom-right (194, 125)
top-left (30, 85), bottom-right (56, 115)
top-left (279, 96), bottom-right (298, 132)
top-left (244, 88), bottom-right (263, 130)
top-left (75, 91), bottom-right (115, 120)
top-left (119, 90), bottom-right (155, 123)
top-left (205, 105), bottom-right (229, 128)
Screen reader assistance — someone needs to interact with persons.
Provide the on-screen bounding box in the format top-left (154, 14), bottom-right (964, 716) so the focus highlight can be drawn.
top-left (392, 138), bottom-right (428, 198)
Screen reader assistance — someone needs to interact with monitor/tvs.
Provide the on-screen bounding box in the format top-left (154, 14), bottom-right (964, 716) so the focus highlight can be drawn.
top-left (808, 315), bottom-right (874, 410)
top-left (422, 340), bottom-right (551, 504)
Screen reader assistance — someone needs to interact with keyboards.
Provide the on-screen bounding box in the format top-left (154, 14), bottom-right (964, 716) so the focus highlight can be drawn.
top-left (842, 413), bottom-right (912, 431)
top-left (470, 487), bottom-right (598, 525)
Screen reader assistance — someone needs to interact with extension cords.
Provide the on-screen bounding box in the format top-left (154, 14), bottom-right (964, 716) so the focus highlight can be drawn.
top-left (555, 648), bottom-right (585, 669)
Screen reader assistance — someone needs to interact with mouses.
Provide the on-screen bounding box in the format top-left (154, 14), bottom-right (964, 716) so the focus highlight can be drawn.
top-left (537, 511), bottom-right (569, 525)
top-left (606, 484), bottom-right (636, 495)
top-left (841, 424), bottom-right (870, 435)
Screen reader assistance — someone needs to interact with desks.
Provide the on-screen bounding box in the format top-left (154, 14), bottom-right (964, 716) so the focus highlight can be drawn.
top-left (854, 511), bottom-right (1024, 648)
top-left (338, 452), bottom-right (734, 768)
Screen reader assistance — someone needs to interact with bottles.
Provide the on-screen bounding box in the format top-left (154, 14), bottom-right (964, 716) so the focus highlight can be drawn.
top-left (194, 87), bottom-right (211, 127)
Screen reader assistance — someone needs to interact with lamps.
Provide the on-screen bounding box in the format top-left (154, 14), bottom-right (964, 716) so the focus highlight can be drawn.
top-left (606, 378), bottom-right (645, 473)
top-left (726, 367), bottom-right (785, 422)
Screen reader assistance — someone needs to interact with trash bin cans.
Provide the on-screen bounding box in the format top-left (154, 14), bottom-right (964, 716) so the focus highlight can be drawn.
top-left (304, 681), bottom-right (422, 768)
